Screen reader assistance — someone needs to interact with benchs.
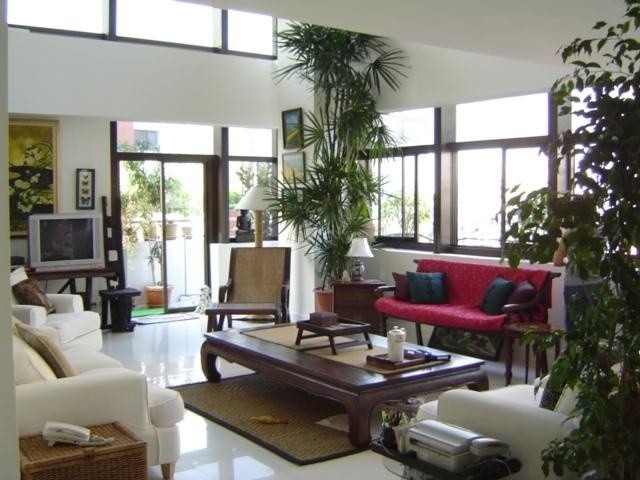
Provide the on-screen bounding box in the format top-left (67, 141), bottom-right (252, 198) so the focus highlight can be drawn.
top-left (370, 258), bottom-right (564, 387)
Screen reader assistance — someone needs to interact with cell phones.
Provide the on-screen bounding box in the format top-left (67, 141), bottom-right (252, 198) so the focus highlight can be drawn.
top-left (433, 353), bottom-right (452, 362)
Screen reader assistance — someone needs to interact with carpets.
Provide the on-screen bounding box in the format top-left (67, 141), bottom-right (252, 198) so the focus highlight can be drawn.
top-left (128, 310), bottom-right (200, 327)
top-left (126, 301), bottom-right (182, 318)
top-left (162, 369), bottom-right (387, 471)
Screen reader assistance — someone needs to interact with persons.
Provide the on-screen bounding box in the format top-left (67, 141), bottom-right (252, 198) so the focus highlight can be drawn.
top-left (552, 206), bottom-right (604, 379)
top-left (237, 210), bottom-right (251, 232)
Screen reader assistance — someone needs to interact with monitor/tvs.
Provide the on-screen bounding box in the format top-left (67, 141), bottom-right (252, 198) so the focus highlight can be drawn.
top-left (28, 212), bottom-right (107, 272)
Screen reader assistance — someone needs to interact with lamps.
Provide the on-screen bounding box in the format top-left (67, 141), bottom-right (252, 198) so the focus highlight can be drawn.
top-left (234, 183), bottom-right (282, 249)
top-left (343, 234), bottom-right (377, 281)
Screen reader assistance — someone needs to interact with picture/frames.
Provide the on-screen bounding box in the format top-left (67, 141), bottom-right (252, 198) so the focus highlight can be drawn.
top-left (9, 118), bottom-right (63, 241)
top-left (281, 150), bottom-right (308, 187)
top-left (76, 167), bottom-right (96, 211)
top-left (280, 107), bottom-right (306, 150)
top-left (280, 187), bottom-right (308, 223)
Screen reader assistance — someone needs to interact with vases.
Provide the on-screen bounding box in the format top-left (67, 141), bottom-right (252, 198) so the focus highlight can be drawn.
top-left (181, 227), bottom-right (193, 240)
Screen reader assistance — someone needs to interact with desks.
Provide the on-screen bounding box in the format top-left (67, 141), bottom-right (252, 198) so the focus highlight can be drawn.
top-left (28, 265), bottom-right (118, 332)
top-left (373, 441), bottom-right (525, 480)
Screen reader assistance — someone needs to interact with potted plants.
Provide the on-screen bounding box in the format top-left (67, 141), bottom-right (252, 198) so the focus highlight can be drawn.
top-left (161, 217), bottom-right (178, 242)
top-left (118, 137), bottom-right (193, 308)
top-left (262, 20), bottom-right (413, 317)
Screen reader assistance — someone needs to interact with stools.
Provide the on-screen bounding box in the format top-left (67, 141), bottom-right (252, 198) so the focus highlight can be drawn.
top-left (97, 286), bottom-right (143, 333)
top-left (500, 320), bottom-right (560, 390)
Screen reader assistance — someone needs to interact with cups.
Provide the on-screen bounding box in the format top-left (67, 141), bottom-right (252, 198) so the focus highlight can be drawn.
top-left (383, 422), bottom-right (410, 454)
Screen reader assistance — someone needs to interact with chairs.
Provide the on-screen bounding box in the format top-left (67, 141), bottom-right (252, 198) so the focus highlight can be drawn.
top-left (11, 266), bottom-right (104, 354)
top-left (10, 304), bottom-right (187, 480)
top-left (203, 246), bottom-right (294, 335)
top-left (409, 319), bottom-right (640, 480)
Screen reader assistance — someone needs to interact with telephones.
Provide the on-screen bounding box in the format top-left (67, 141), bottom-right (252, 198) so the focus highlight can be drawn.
top-left (470, 437), bottom-right (509, 456)
top-left (43, 422), bottom-right (90, 444)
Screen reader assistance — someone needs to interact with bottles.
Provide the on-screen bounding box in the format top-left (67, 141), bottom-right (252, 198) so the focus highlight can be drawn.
top-left (386, 325), bottom-right (407, 364)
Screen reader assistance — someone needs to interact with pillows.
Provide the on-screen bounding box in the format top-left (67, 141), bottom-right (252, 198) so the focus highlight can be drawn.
top-left (480, 275), bottom-right (515, 317)
top-left (404, 269), bottom-right (450, 305)
top-left (11, 277), bottom-right (58, 317)
top-left (507, 278), bottom-right (537, 308)
top-left (391, 269), bottom-right (412, 303)
top-left (13, 320), bottom-right (76, 380)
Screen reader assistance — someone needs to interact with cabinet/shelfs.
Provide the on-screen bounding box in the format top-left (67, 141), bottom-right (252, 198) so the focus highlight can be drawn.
top-left (208, 240), bottom-right (293, 310)
top-left (331, 277), bottom-right (386, 339)
